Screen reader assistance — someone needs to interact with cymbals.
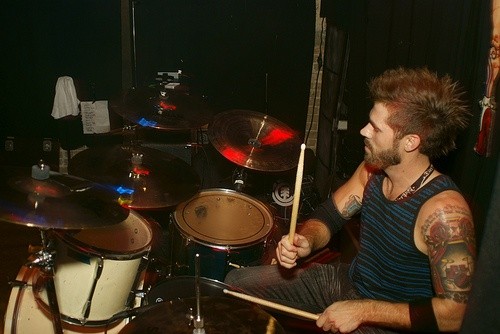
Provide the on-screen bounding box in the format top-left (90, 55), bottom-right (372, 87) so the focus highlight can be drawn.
top-left (110, 97), bottom-right (212, 129)
top-left (209, 109), bottom-right (303, 173)
top-left (66, 141), bottom-right (200, 207)
top-left (2, 166), bottom-right (130, 229)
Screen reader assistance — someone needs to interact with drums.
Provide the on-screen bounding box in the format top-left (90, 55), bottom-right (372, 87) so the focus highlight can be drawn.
top-left (141, 276), bottom-right (235, 302)
top-left (174, 186), bottom-right (274, 283)
top-left (28, 210), bottom-right (154, 326)
top-left (118, 296), bottom-right (284, 333)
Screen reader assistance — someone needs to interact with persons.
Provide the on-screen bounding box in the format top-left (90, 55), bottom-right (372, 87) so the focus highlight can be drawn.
top-left (224, 66), bottom-right (476, 334)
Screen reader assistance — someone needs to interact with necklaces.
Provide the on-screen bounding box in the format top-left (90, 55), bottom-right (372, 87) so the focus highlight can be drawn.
top-left (394, 163), bottom-right (435, 201)
